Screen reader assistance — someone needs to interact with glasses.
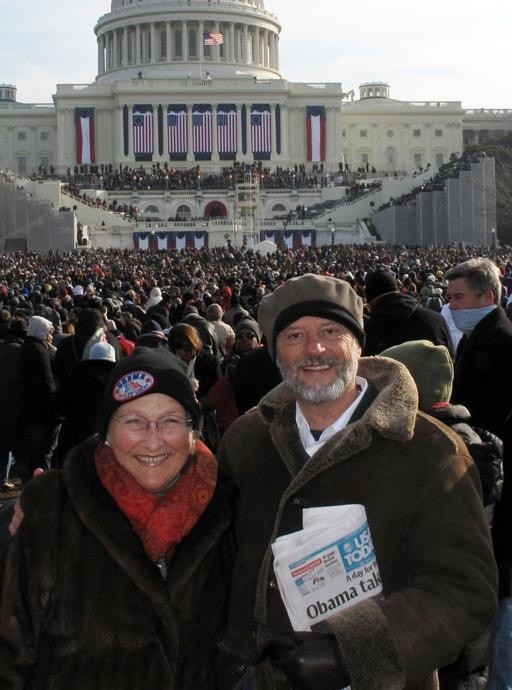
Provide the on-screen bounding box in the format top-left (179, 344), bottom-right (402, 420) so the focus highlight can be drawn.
top-left (236, 332), bottom-right (257, 340)
top-left (114, 411), bottom-right (194, 437)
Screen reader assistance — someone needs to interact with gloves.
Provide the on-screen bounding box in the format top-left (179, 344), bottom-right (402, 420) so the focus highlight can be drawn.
top-left (271, 631), bottom-right (350, 690)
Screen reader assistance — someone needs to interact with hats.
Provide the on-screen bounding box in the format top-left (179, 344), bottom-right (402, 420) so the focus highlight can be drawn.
top-left (89, 341), bottom-right (116, 363)
top-left (403, 259), bottom-right (449, 295)
top-left (141, 319), bottom-right (163, 334)
top-left (96, 346), bottom-right (201, 444)
top-left (378, 339), bottom-right (455, 412)
top-left (365, 271), bottom-right (400, 306)
top-left (233, 315), bottom-right (263, 344)
top-left (257, 274), bottom-right (366, 366)
top-left (27, 316), bottom-right (55, 341)
top-left (8, 317), bottom-right (28, 332)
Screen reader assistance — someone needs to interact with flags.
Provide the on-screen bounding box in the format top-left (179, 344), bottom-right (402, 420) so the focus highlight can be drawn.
top-left (203, 31), bottom-right (224, 45)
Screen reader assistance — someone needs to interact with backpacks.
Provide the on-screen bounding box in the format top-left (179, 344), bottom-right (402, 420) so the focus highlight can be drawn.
top-left (429, 296), bottom-right (440, 311)
